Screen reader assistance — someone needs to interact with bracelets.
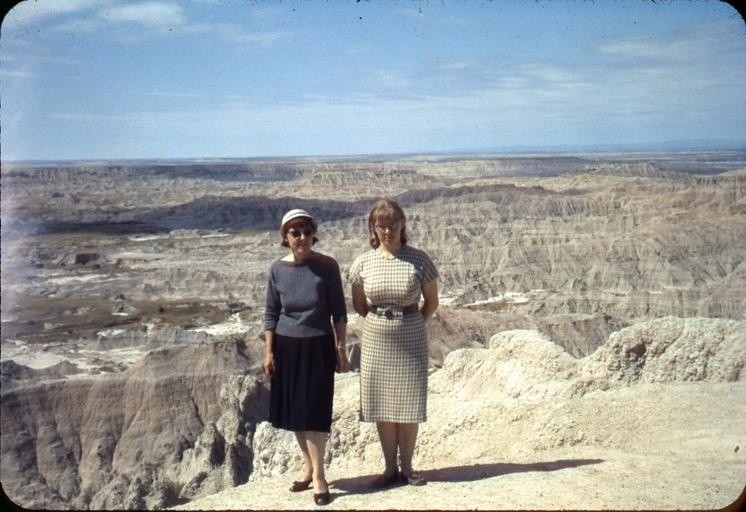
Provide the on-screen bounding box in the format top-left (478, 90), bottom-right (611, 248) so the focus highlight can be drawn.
top-left (337, 345), bottom-right (347, 350)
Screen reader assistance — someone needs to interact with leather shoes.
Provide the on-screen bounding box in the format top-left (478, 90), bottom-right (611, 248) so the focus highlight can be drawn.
top-left (400, 470), bottom-right (427, 486)
top-left (288, 479), bottom-right (312, 492)
top-left (371, 469), bottom-right (399, 489)
top-left (313, 480), bottom-right (330, 506)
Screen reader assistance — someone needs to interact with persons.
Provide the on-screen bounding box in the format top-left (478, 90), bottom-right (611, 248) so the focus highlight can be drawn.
top-left (259, 207), bottom-right (350, 507)
top-left (346, 199), bottom-right (443, 489)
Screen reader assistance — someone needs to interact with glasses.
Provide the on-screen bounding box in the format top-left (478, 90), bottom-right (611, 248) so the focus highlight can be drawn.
top-left (374, 220), bottom-right (399, 234)
top-left (287, 229), bottom-right (312, 238)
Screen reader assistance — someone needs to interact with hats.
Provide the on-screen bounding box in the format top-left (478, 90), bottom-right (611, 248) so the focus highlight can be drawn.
top-left (281, 208), bottom-right (312, 226)
